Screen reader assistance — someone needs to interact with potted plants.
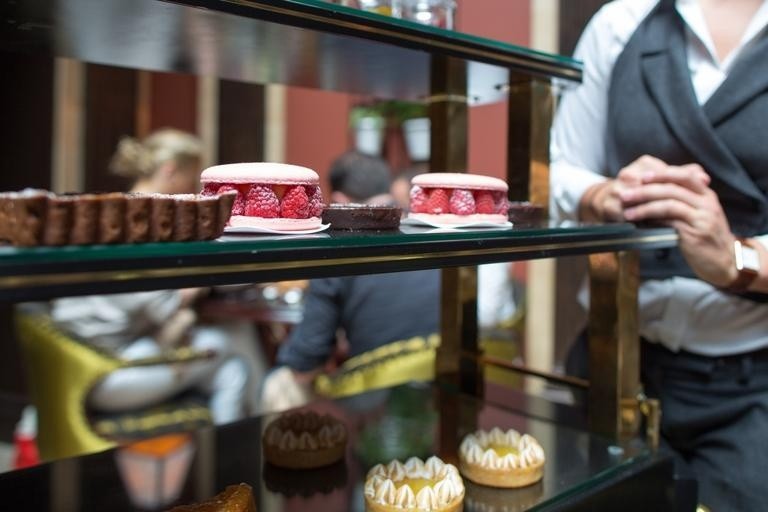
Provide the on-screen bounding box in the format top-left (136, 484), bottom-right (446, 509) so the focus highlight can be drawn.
top-left (397, 102), bottom-right (432, 162)
top-left (346, 104), bottom-right (387, 155)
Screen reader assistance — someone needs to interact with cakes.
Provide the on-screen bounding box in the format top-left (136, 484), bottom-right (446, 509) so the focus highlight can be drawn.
top-left (363, 454), bottom-right (466, 512)
top-left (196, 160), bottom-right (328, 236)
top-left (0, 186), bottom-right (237, 247)
top-left (261, 408), bottom-right (348, 467)
top-left (166, 481), bottom-right (258, 511)
top-left (457, 426), bottom-right (546, 488)
top-left (406, 172), bottom-right (511, 225)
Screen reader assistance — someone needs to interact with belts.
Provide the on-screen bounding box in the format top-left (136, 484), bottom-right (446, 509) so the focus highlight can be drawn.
top-left (641, 341), bottom-right (764, 375)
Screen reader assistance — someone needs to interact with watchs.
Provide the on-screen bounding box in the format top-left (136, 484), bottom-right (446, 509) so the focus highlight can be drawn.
top-left (717, 237), bottom-right (758, 296)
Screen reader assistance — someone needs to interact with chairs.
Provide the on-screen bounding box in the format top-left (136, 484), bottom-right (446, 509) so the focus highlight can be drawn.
top-left (16, 314), bottom-right (219, 512)
top-left (314, 335), bottom-right (440, 397)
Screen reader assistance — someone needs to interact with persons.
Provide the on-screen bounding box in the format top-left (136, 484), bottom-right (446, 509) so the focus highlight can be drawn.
top-left (391, 162), bottom-right (477, 355)
top-left (52, 128), bottom-right (267, 425)
top-left (546, 0), bottom-right (766, 512)
top-left (278, 150), bottom-right (442, 402)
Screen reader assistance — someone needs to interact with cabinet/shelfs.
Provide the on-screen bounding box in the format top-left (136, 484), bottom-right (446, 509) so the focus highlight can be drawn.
top-left (1, 0), bottom-right (680, 512)
